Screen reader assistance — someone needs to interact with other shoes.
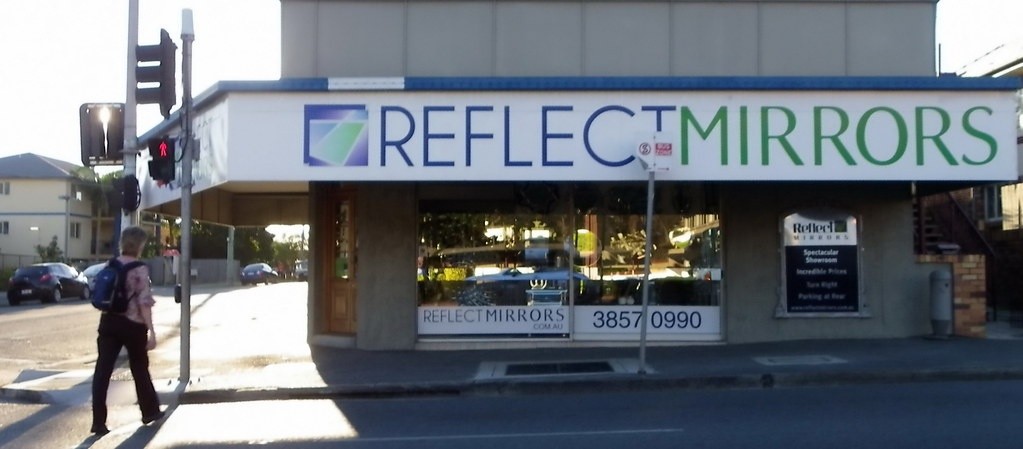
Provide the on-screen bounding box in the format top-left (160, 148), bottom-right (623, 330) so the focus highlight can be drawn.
top-left (91, 423), bottom-right (112, 436)
top-left (142, 409), bottom-right (164, 424)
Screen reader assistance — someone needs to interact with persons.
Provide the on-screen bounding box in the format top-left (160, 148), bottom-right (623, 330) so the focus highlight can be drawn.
top-left (89, 227), bottom-right (165, 433)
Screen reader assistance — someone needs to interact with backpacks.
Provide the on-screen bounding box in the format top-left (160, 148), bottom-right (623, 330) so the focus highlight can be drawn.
top-left (91, 258), bottom-right (148, 312)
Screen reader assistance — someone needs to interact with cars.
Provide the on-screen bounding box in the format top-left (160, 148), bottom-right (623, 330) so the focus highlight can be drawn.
top-left (240, 263), bottom-right (279, 286)
top-left (83, 261), bottom-right (153, 298)
top-left (7, 261), bottom-right (80, 306)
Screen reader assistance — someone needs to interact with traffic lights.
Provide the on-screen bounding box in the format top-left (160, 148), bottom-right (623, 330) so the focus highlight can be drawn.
top-left (147, 137), bottom-right (176, 180)
top-left (133, 28), bottom-right (176, 119)
